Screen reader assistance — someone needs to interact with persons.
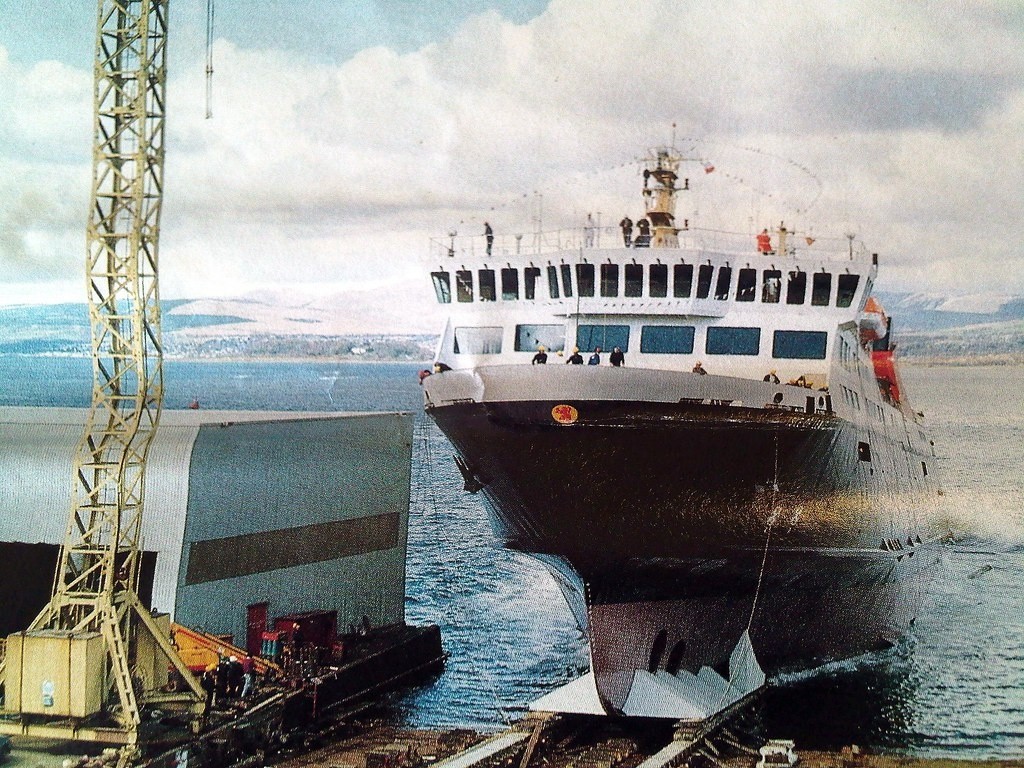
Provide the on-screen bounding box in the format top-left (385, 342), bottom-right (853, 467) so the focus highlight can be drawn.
top-left (565, 347), bottom-right (586, 365)
top-left (762, 369), bottom-right (782, 385)
top-left (609, 345), bottom-right (626, 368)
top-left (635, 213), bottom-right (650, 237)
top-left (479, 221), bottom-right (495, 255)
top-left (531, 345), bottom-right (551, 365)
top-left (413, 369), bottom-right (433, 386)
top-left (200, 652), bottom-right (257, 715)
top-left (618, 214), bottom-right (635, 249)
top-left (589, 346), bottom-right (602, 366)
top-left (433, 362), bottom-right (455, 372)
top-left (581, 212), bottom-right (599, 249)
top-left (754, 228), bottom-right (775, 256)
top-left (692, 360), bottom-right (710, 375)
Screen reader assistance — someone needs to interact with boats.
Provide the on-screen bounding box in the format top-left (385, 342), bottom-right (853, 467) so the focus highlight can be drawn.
top-left (420, 123), bottom-right (938, 716)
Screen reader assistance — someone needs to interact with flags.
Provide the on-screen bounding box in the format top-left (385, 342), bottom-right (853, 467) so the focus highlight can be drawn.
top-left (703, 161), bottom-right (714, 173)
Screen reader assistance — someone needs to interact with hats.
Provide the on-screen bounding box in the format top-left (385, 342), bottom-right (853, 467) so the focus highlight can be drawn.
top-left (557, 351), bottom-right (563, 356)
top-left (696, 360), bottom-right (702, 366)
top-left (205, 664), bottom-right (213, 672)
top-left (538, 346), bottom-right (545, 351)
top-left (572, 347), bottom-right (579, 353)
top-left (229, 656), bottom-right (237, 662)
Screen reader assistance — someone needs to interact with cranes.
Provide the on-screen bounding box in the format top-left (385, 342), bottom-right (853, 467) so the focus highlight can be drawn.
top-left (0, 0), bottom-right (214, 745)
